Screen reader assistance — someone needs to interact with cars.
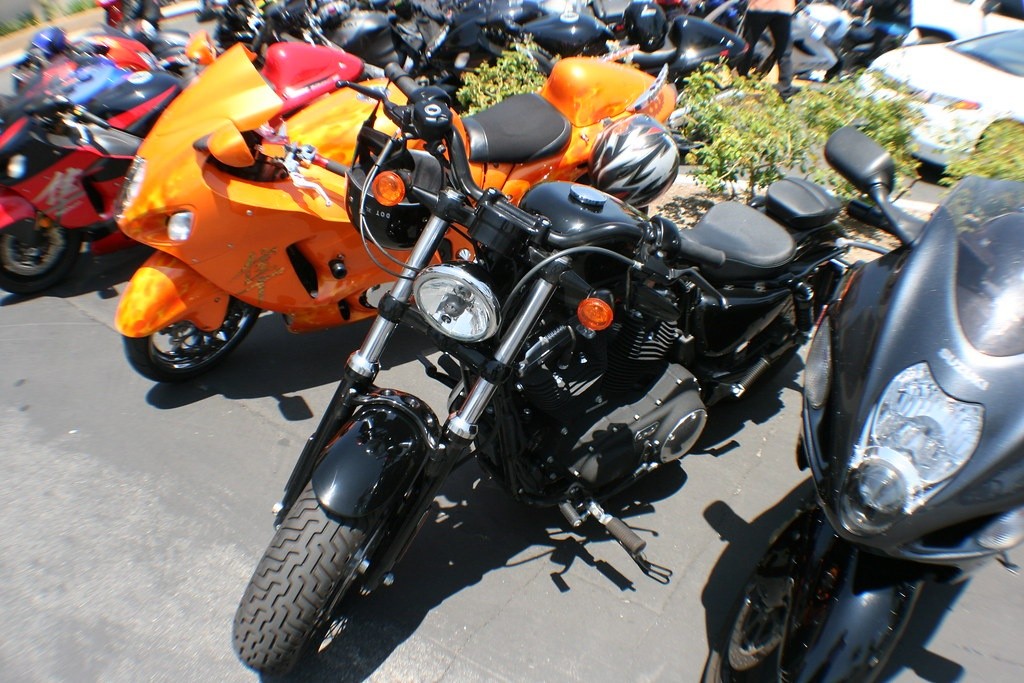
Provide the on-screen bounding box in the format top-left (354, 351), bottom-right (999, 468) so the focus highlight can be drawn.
top-left (858, 26), bottom-right (1024, 166)
top-left (912, 0), bottom-right (1024, 42)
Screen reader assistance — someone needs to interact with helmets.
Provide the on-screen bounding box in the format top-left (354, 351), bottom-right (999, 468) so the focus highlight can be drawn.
top-left (31, 26), bottom-right (65, 57)
top-left (622, 1), bottom-right (668, 53)
top-left (587, 113), bottom-right (680, 210)
top-left (121, 18), bottom-right (158, 49)
top-left (317, 0), bottom-right (351, 31)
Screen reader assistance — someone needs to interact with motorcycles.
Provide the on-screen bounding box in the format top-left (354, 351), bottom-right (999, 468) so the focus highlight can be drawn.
top-left (702, 127), bottom-right (1024, 683)
top-left (1, 0), bottom-right (924, 676)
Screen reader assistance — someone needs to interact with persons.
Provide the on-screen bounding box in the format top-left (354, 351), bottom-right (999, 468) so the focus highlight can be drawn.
top-left (390, 0), bottom-right (451, 69)
top-left (740, 0), bottom-right (802, 98)
top-left (840, 0), bottom-right (914, 58)
top-left (94, 0), bottom-right (124, 30)
